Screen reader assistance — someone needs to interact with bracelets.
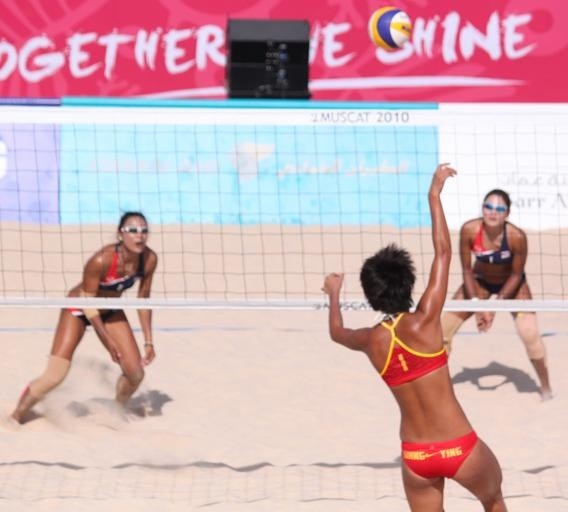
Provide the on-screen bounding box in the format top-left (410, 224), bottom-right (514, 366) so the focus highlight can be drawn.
top-left (144, 339), bottom-right (154, 345)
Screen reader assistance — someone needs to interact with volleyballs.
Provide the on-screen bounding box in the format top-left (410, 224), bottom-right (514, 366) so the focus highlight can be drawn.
top-left (369, 6), bottom-right (412, 51)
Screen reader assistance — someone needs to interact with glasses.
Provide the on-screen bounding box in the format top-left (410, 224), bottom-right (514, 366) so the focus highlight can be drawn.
top-left (119, 226), bottom-right (149, 235)
top-left (483, 203), bottom-right (508, 214)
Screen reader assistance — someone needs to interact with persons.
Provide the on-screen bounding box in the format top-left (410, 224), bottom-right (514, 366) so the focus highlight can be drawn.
top-left (12, 210), bottom-right (158, 428)
top-left (321, 161), bottom-right (505, 510)
top-left (439, 190), bottom-right (554, 399)
